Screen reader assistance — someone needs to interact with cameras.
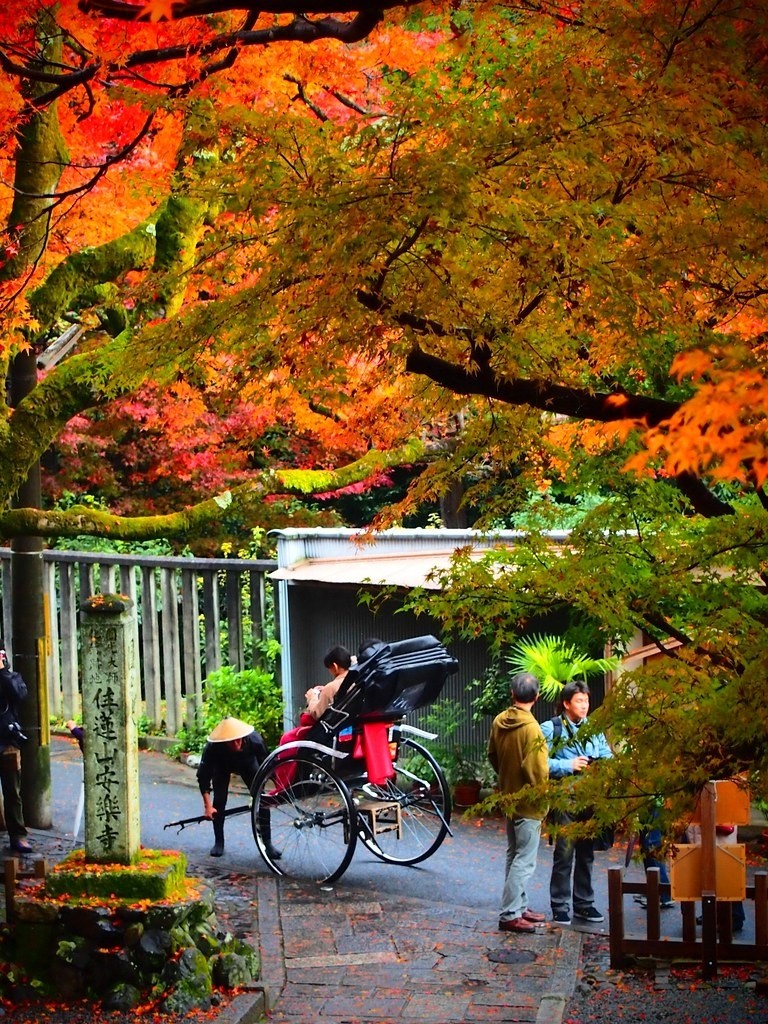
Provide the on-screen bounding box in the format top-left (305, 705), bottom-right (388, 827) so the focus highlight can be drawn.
top-left (586, 755), bottom-right (603, 766)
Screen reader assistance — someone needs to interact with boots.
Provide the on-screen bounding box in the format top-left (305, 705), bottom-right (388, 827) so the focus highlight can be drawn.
top-left (258, 808), bottom-right (283, 858)
top-left (210, 812), bottom-right (225, 856)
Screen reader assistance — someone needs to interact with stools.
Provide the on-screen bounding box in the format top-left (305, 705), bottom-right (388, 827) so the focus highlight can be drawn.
top-left (342, 800), bottom-right (402, 845)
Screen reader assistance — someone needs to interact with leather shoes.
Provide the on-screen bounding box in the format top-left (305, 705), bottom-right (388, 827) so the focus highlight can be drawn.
top-left (499, 918), bottom-right (535, 933)
top-left (522, 908), bottom-right (545, 922)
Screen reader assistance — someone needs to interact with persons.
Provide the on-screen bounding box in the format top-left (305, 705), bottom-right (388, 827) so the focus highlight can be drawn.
top-left (682, 824), bottom-right (746, 933)
top-left (540, 680), bottom-right (615, 926)
top-left (632, 793), bottom-right (675, 908)
top-left (0, 635), bottom-right (33, 854)
top-left (269, 645), bottom-right (356, 795)
top-left (485, 673), bottom-right (550, 932)
top-left (196, 717), bottom-right (283, 859)
top-left (66, 720), bottom-right (84, 754)
top-left (350, 638), bottom-right (382, 666)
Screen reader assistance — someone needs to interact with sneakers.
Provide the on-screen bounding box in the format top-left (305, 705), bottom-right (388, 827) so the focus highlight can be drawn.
top-left (573, 907), bottom-right (604, 921)
top-left (552, 912), bottom-right (571, 925)
top-left (633, 895), bottom-right (643, 900)
top-left (641, 899), bottom-right (675, 907)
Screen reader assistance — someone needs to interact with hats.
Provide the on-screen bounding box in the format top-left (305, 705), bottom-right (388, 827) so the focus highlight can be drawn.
top-left (207, 716), bottom-right (254, 742)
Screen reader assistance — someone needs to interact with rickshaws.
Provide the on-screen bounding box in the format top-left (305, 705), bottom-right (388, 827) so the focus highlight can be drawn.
top-left (163, 635), bottom-right (459, 885)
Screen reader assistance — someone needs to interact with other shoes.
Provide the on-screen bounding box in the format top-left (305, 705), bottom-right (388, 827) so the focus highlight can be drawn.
top-left (10, 837), bottom-right (32, 853)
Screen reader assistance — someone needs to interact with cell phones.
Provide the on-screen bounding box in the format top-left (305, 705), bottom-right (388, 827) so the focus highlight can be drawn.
top-left (313, 688), bottom-right (320, 695)
